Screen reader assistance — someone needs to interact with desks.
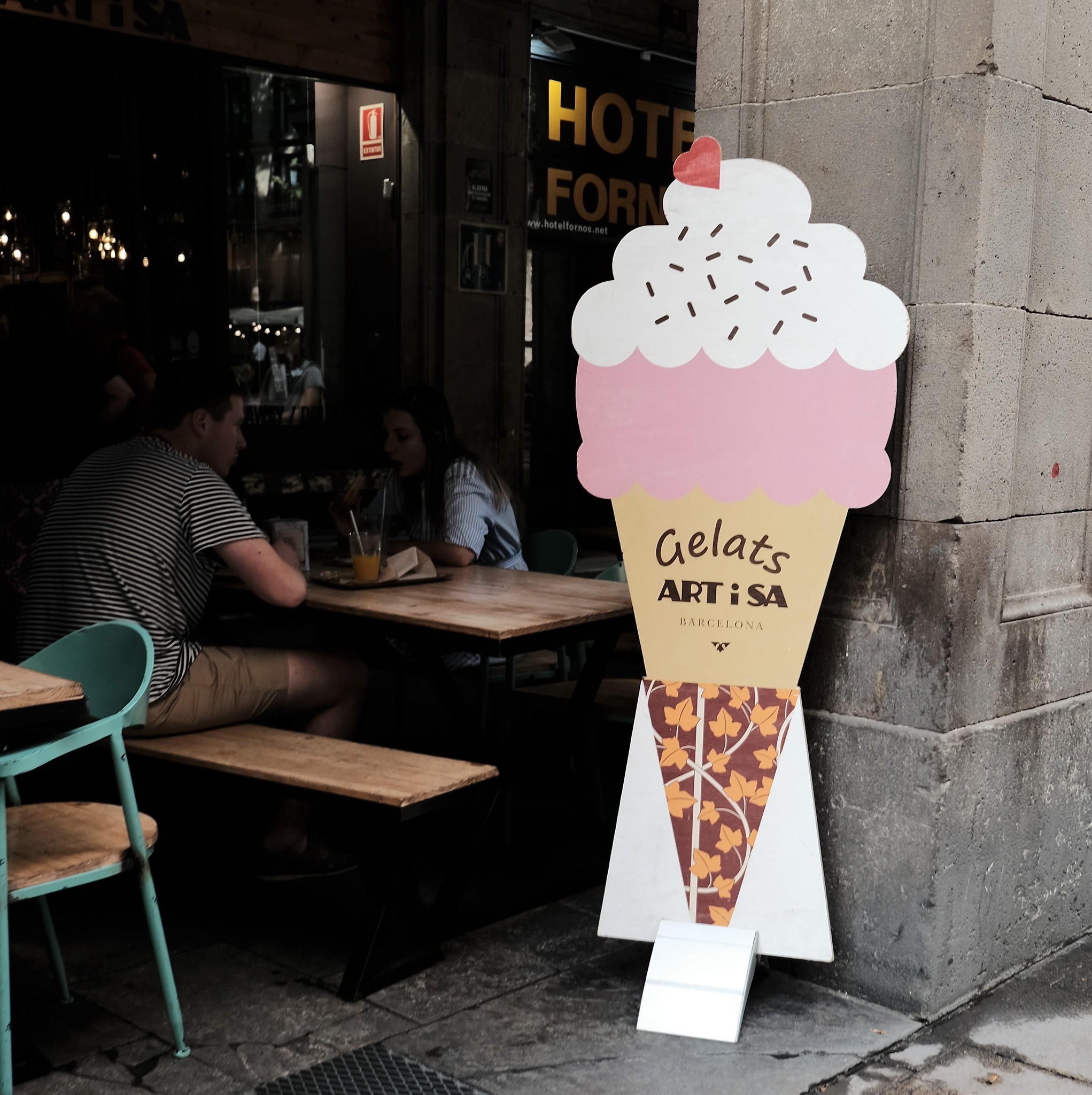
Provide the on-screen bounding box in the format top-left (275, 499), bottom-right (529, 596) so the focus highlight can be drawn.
top-left (0, 657), bottom-right (88, 1095)
top-left (214, 556), bottom-right (634, 845)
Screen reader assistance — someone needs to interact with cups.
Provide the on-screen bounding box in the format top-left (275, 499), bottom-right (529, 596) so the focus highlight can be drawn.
top-left (348, 531), bottom-right (382, 582)
top-left (367, 530), bottom-right (389, 580)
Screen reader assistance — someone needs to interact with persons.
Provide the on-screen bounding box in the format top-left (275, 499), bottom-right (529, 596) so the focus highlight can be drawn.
top-left (11, 358), bottom-right (370, 881)
top-left (0, 273), bottom-right (328, 437)
top-left (329, 380), bottom-right (526, 754)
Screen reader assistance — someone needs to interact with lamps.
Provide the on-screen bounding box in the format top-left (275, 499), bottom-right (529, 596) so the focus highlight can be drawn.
top-left (530, 29), bottom-right (576, 54)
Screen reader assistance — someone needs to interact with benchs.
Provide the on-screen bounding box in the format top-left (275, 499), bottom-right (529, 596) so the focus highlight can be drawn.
top-left (91, 676), bottom-right (642, 1003)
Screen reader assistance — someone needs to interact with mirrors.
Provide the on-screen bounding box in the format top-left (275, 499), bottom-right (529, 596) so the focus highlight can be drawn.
top-left (220, 64), bottom-right (403, 535)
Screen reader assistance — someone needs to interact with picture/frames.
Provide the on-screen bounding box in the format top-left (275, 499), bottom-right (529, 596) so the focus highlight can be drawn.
top-left (457, 220), bottom-right (509, 295)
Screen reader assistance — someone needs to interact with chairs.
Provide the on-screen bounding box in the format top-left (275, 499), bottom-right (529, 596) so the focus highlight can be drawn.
top-left (0, 617), bottom-right (191, 1059)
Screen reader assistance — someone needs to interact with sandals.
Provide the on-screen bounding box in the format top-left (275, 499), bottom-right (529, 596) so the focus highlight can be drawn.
top-left (256, 837), bottom-right (357, 883)
